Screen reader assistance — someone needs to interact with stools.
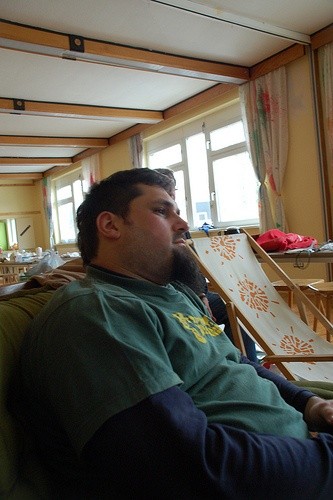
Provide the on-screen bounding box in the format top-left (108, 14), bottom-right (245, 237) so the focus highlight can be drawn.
top-left (269, 278), bottom-right (327, 328)
top-left (305, 281), bottom-right (333, 343)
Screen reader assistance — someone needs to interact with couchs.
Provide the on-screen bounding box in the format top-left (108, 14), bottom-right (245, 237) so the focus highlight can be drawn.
top-left (0, 257), bottom-right (333, 500)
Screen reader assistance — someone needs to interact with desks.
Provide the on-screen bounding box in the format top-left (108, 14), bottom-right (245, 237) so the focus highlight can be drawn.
top-left (0, 262), bottom-right (37, 286)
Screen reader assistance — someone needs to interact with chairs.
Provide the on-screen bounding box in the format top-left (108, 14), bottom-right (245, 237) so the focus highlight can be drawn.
top-left (180, 227), bottom-right (333, 383)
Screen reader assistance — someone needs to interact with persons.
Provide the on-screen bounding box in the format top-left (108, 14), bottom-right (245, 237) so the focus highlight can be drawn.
top-left (11, 167), bottom-right (333, 500)
top-left (152, 168), bottom-right (260, 364)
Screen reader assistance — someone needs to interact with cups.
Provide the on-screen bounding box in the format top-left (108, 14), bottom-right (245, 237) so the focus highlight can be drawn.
top-left (35, 247), bottom-right (42, 258)
